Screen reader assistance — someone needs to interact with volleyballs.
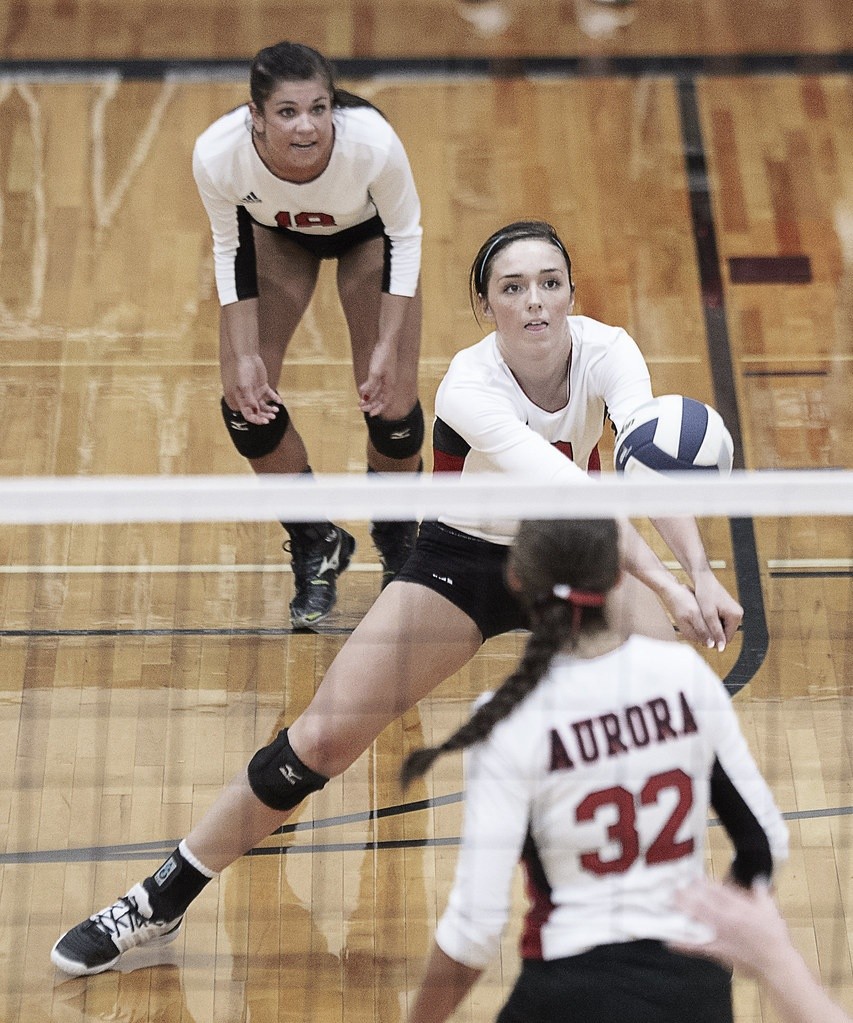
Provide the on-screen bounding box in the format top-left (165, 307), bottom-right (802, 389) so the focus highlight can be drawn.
top-left (612, 393), bottom-right (736, 477)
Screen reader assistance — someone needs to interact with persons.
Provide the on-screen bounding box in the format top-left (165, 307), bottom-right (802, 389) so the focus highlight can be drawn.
top-left (192, 39), bottom-right (421, 646)
top-left (48, 221), bottom-right (743, 979)
top-left (375, 516), bottom-right (853, 1023)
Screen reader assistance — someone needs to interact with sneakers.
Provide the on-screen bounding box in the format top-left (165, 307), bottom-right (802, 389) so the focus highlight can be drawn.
top-left (49, 877), bottom-right (183, 976)
top-left (281, 521), bottom-right (356, 629)
top-left (369, 521), bottom-right (419, 592)
